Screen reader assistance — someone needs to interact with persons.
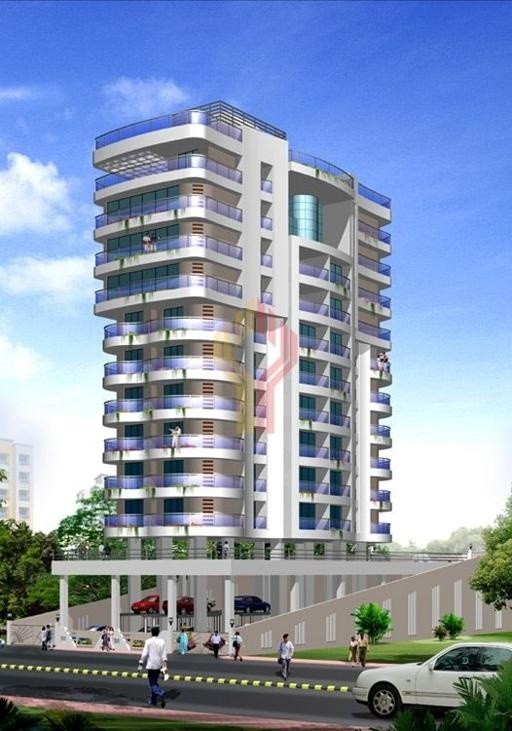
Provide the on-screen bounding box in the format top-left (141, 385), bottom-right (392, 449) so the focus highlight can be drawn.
top-left (150, 233), bottom-right (160, 252)
top-left (143, 231), bottom-right (151, 253)
top-left (231, 633), bottom-right (243, 661)
top-left (278, 633), bottom-right (295, 681)
top-left (46, 624), bottom-right (52, 650)
top-left (377, 353), bottom-right (384, 372)
top-left (209, 541), bottom-right (217, 559)
top-left (178, 629), bottom-right (188, 656)
top-left (101, 630), bottom-right (111, 652)
top-left (211, 630), bottom-right (221, 659)
top-left (138, 626), bottom-right (169, 706)
top-left (41, 626), bottom-right (48, 650)
top-left (222, 540), bottom-right (230, 559)
top-left (468, 545), bottom-right (474, 559)
top-left (382, 354), bottom-right (391, 372)
top-left (349, 636), bottom-right (359, 665)
top-left (169, 426), bottom-right (182, 449)
top-left (358, 633), bottom-right (367, 668)
top-left (217, 542), bottom-right (223, 559)
top-left (103, 543), bottom-right (112, 559)
top-left (368, 546), bottom-right (376, 562)
top-left (99, 542), bottom-right (104, 560)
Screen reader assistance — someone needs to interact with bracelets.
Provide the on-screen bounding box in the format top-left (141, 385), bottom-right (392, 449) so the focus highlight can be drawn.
top-left (139, 660), bottom-right (143, 664)
